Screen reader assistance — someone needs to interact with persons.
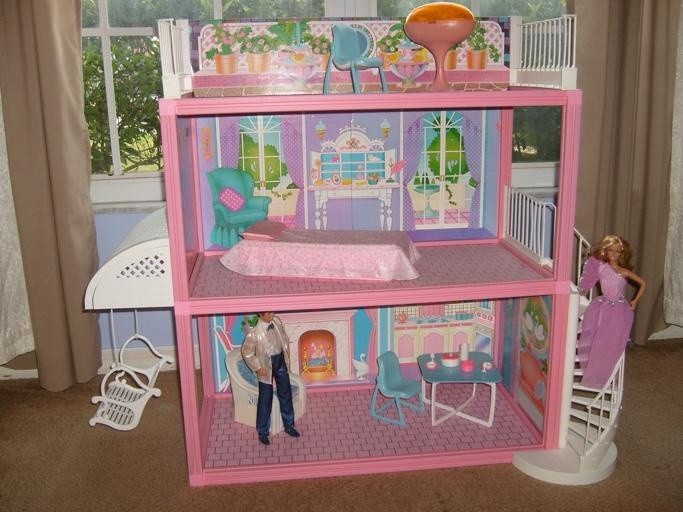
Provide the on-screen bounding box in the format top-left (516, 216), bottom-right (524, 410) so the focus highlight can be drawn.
top-left (578, 234), bottom-right (644, 391)
top-left (242, 311), bottom-right (300, 445)
